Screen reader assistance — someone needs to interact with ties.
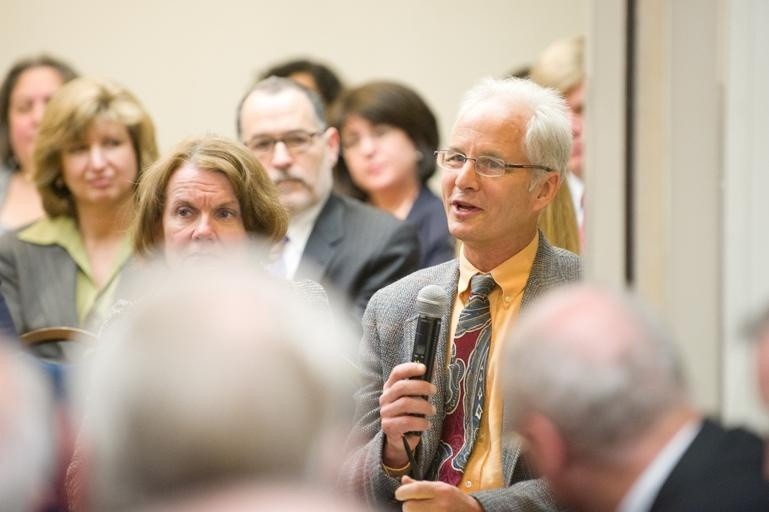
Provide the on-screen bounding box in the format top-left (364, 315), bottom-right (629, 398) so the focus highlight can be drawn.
top-left (432, 273), bottom-right (500, 486)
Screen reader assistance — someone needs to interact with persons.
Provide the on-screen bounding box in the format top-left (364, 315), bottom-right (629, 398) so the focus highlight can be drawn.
top-left (497, 281), bottom-right (769, 512)
top-left (534, 32), bottom-right (586, 256)
top-left (342, 77), bottom-right (596, 512)
top-left (2, 331), bottom-right (60, 510)
top-left (2, 52), bottom-right (455, 342)
top-left (51, 254), bottom-right (370, 511)
top-left (738, 307), bottom-right (769, 417)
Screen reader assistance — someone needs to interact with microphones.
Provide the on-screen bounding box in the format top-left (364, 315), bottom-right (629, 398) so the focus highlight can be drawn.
top-left (404, 284), bottom-right (451, 437)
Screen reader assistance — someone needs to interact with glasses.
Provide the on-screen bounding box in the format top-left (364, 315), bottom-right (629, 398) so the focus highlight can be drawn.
top-left (433, 150), bottom-right (552, 177)
top-left (243, 125), bottom-right (330, 158)
